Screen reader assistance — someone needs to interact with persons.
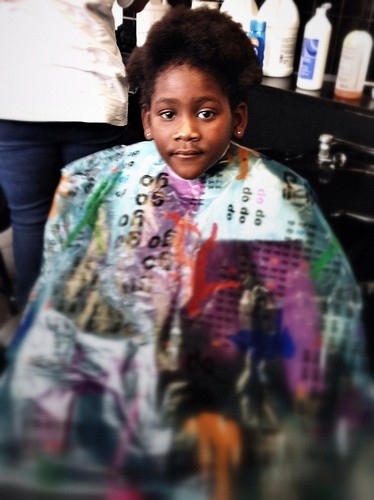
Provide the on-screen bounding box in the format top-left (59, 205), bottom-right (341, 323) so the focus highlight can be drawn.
top-left (124, 5), bottom-right (261, 179)
top-left (0, 0), bottom-right (150, 306)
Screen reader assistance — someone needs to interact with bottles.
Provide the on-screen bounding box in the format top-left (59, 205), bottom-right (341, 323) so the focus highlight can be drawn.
top-left (334, 19), bottom-right (373, 99)
top-left (220, 0), bottom-right (258, 39)
top-left (296, 4), bottom-right (331, 90)
top-left (256, 0), bottom-right (299, 78)
top-left (250, 20), bottom-right (266, 69)
top-left (137, 0), bottom-right (172, 48)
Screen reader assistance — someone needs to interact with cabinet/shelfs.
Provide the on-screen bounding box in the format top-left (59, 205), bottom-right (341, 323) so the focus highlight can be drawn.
top-left (113, 42), bottom-right (374, 345)
